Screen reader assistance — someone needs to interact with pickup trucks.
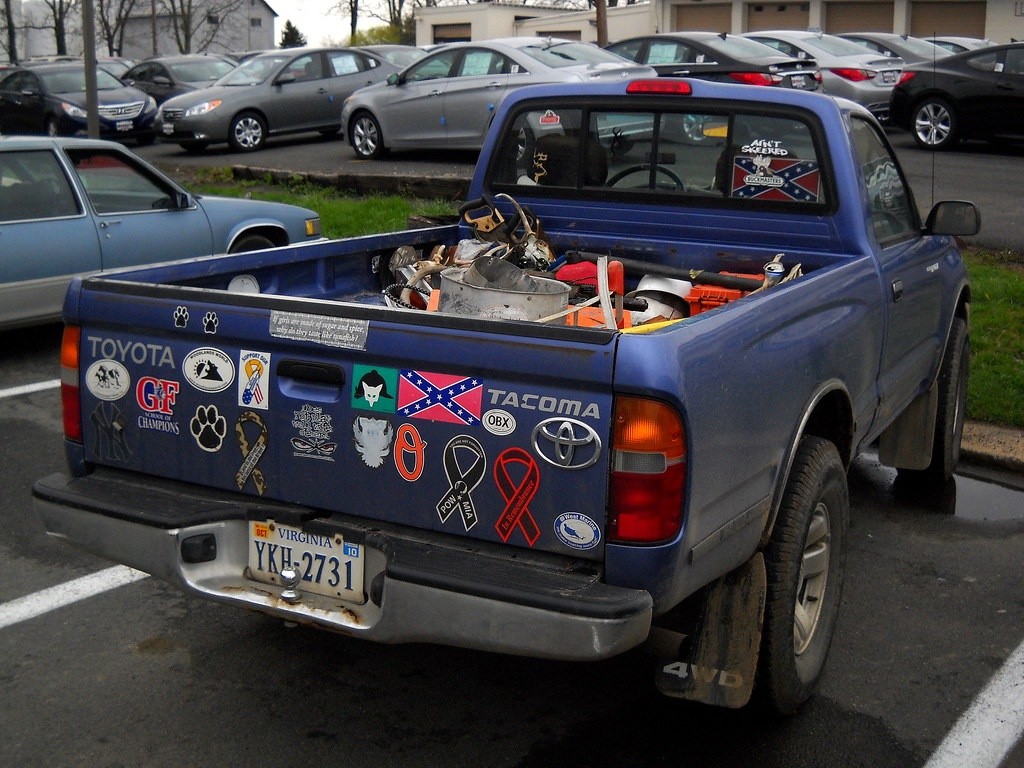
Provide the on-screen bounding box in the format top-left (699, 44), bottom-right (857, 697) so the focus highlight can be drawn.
top-left (26, 74), bottom-right (980, 715)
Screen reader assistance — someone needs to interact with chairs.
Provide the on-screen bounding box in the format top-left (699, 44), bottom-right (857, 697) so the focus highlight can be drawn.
top-left (526, 134), bottom-right (609, 187)
top-left (716, 143), bottom-right (797, 203)
top-left (295, 62), bottom-right (321, 80)
top-left (272, 62), bottom-right (291, 75)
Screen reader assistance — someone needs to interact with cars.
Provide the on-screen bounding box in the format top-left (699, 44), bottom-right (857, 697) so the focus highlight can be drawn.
top-left (833, 31), bottom-right (960, 66)
top-left (155, 46), bottom-right (422, 153)
top-left (338, 37), bottom-right (665, 160)
top-left (0, 47), bottom-right (490, 140)
top-left (887, 39), bottom-right (1024, 152)
top-left (0, 132), bottom-right (332, 334)
top-left (598, 29), bottom-right (826, 137)
top-left (741, 32), bottom-right (907, 119)
top-left (920, 36), bottom-right (1003, 70)
top-left (0, 64), bottom-right (162, 147)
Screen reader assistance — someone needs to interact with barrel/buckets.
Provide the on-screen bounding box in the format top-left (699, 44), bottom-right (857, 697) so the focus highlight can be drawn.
top-left (464, 255), bottom-right (543, 294)
top-left (438, 267), bottom-right (572, 326)
